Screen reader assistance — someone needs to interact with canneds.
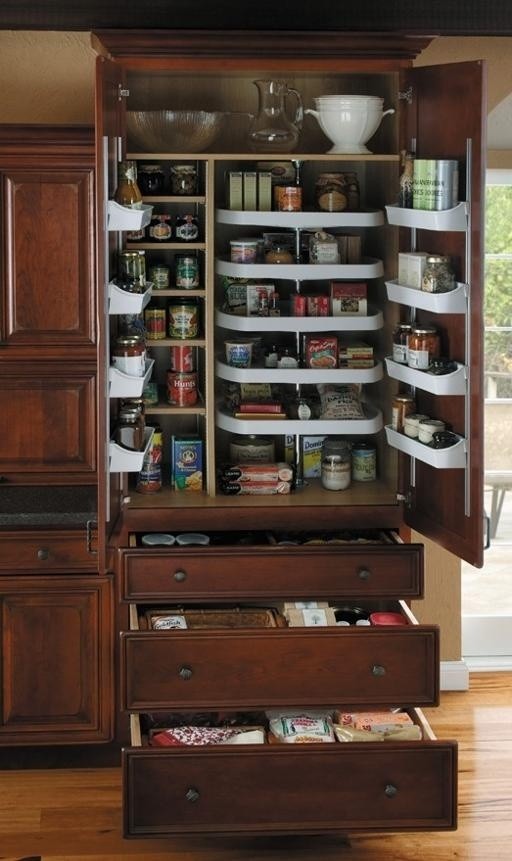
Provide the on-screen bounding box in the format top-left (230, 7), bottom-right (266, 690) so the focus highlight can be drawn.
top-left (127, 231), bottom-right (146, 242)
top-left (322, 438), bottom-right (349, 492)
top-left (176, 255), bottom-right (199, 290)
top-left (144, 308), bottom-right (166, 340)
top-left (405, 414), bottom-right (430, 439)
top-left (351, 438), bottom-right (377, 482)
top-left (275, 186), bottom-right (302, 212)
top-left (393, 323), bottom-right (412, 365)
top-left (229, 240), bottom-right (257, 263)
top-left (169, 164), bottom-right (199, 196)
top-left (120, 398), bottom-right (145, 412)
top-left (111, 335), bottom-right (146, 377)
top-left (118, 313), bottom-right (145, 338)
top-left (342, 172), bottom-right (360, 210)
top-left (139, 164), bottom-right (166, 195)
top-left (418, 419), bottom-right (446, 444)
top-left (149, 215), bottom-right (172, 241)
top-left (256, 238), bottom-right (265, 264)
top-left (409, 327), bottom-right (440, 372)
top-left (148, 261), bottom-right (170, 290)
top-left (168, 298), bottom-right (200, 340)
top-left (176, 214), bottom-right (200, 242)
top-left (392, 396), bottom-right (417, 433)
top-left (166, 371), bottom-right (199, 407)
top-left (170, 346), bottom-right (195, 373)
top-left (314, 171), bottom-right (345, 212)
top-left (118, 409), bottom-right (145, 451)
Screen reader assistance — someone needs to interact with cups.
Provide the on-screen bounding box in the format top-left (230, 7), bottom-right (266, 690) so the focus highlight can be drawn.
top-left (225, 340), bottom-right (254, 370)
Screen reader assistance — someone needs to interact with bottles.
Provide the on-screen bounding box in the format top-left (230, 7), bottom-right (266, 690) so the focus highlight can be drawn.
top-left (258, 292), bottom-right (281, 317)
top-left (399, 151), bottom-right (416, 207)
top-left (115, 159), bottom-right (144, 211)
top-left (354, 440), bottom-right (377, 484)
top-left (321, 438), bottom-right (353, 491)
top-left (315, 170), bottom-right (361, 213)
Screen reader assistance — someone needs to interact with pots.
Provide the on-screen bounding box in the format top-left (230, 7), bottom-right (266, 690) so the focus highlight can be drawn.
top-left (122, 107), bottom-right (255, 153)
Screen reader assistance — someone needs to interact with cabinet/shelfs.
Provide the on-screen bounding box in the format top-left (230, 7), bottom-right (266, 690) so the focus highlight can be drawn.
top-left (90, 30), bottom-right (484, 841)
top-left (0, 122), bottom-right (117, 746)
